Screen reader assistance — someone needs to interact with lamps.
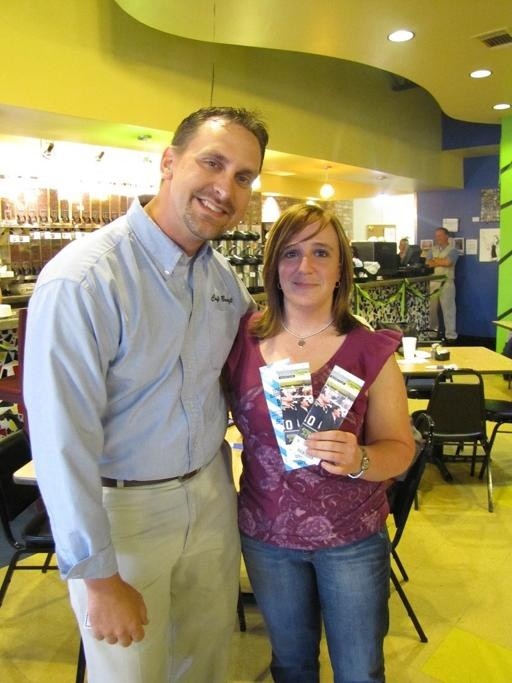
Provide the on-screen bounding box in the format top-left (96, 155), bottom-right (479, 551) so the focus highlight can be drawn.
top-left (321, 165), bottom-right (334, 199)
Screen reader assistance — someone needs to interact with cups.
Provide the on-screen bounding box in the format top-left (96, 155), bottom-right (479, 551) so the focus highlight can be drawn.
top-left (402, 336), bottom-right (417, 359)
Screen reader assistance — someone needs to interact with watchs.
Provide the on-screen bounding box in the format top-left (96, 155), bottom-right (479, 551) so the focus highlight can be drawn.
top-left (348, 446), bottom-right (371, 479)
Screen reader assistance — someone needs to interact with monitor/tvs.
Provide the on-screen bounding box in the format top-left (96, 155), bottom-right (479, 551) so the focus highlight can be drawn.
top-left (402, 244), bottom-right (422, 266)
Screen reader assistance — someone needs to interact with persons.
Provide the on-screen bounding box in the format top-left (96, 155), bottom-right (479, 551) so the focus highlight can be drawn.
top-left (222, 198), bottom-right (420, 682)
top-left (280, 385), bottom-right (345, 433)
top-left (19, 106), bottom-right (269, 682)
top-left (395, 238), bottom-right (420, 271)
top-left (350, 246), bottom-right (364, 268)
top-left (424, 226), bottom-right (460, 344)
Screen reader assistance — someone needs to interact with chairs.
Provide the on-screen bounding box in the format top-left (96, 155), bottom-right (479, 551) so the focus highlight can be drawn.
top-left (388, 414), bottom-right (436, 641)
top-left (405, 329), bottom-right (455, 399)
top-left (455, 338), bottom-right (512, 479)
top-left (0, 429), bottom-right (59, 608)
top-left (412, 368), bottom-right (494, 513)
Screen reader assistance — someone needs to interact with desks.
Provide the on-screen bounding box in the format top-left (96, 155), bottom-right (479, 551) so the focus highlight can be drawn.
top-left (12, 425), bottom-right (258, 608)
top-left (491, 320), bottom-right (512, 331)
top-left (393, 346), bottom-right (512, 462)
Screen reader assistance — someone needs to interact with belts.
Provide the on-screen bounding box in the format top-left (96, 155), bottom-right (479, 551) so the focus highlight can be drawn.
top-left (100, 467), bottom-right (201, 488)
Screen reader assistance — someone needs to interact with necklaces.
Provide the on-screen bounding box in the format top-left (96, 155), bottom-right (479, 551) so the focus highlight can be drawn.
top-left (279, 314), bottom-right (334, 349)
